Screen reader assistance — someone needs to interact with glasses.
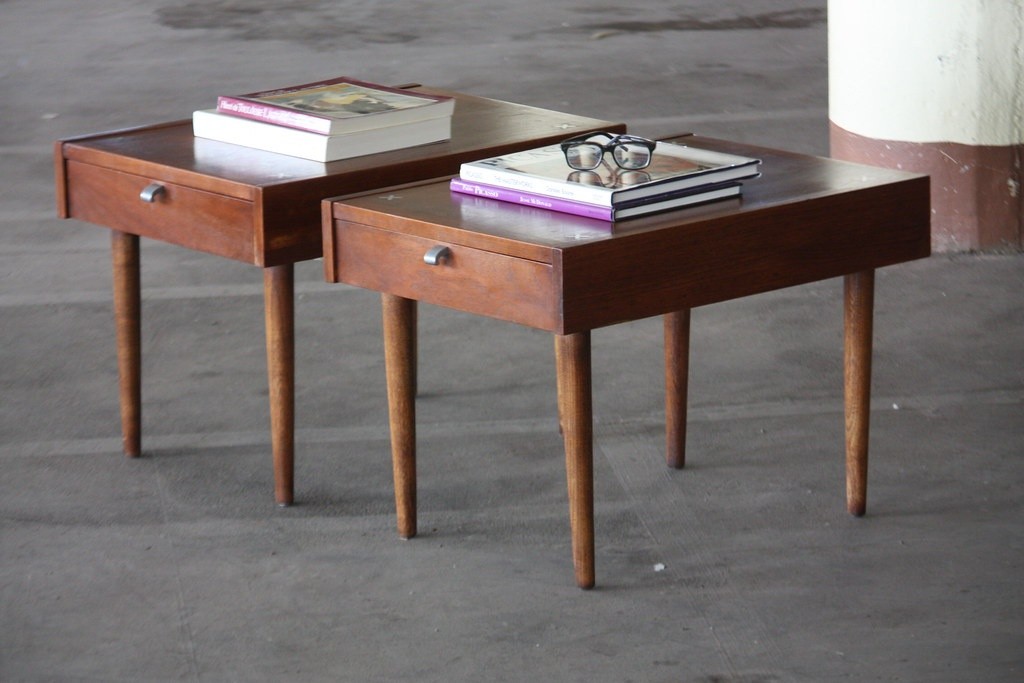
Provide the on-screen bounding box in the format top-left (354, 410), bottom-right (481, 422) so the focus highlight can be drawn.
top-left (567, 157), bottom-right (651, 189)
top-left (560, 130), bottom-right (698, 170)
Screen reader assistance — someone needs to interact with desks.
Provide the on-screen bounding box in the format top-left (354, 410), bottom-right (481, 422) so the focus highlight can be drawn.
top-left (54, 83), bottom-right (630, 510)
top-left (320, 130), bottom-right (933, 590)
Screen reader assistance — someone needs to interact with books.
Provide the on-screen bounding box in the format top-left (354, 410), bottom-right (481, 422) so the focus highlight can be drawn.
top-left (192, 75), bottom-right (455, 161)
top-left (450, 131), bottom-right (763, 221)
